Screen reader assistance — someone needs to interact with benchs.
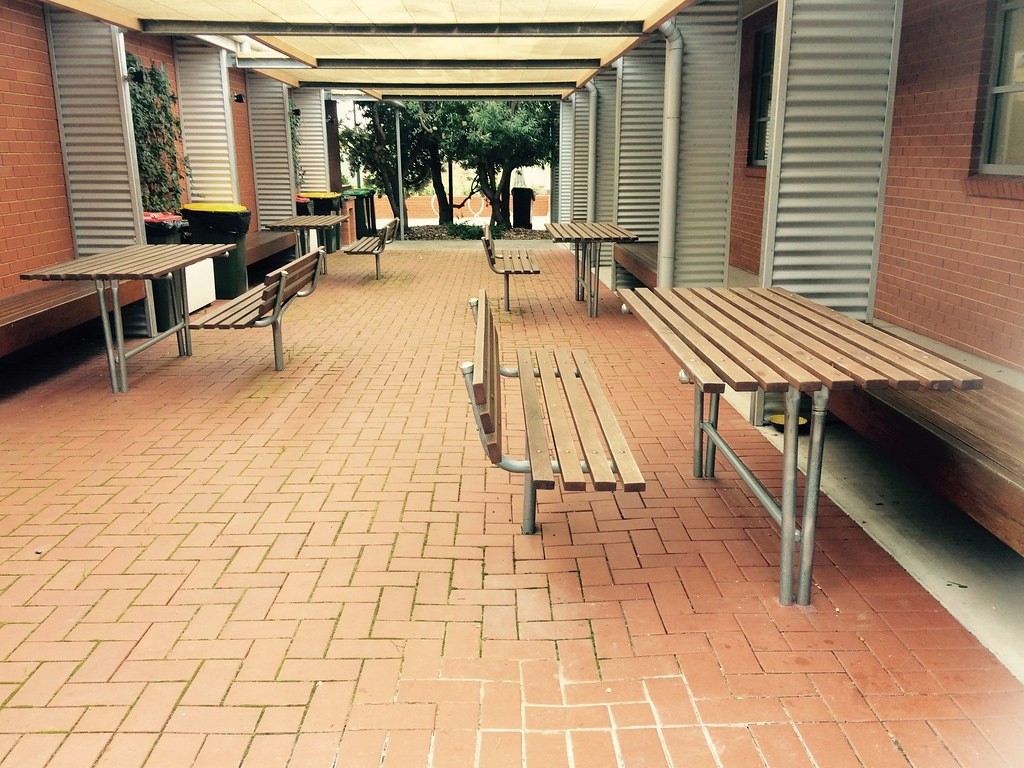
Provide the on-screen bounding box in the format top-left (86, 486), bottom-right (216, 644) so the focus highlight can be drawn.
top-left (481, 224), bottom-right (540, 312)
top-left (345, 215), bottom-right (402, 281)
top-left (242, 230), bottom-right (297, 295)
top-left (189, 246), bottom-right (327, 371)
top-left (800, 386), bottom-right (1024, 560)
top-left (614, 241), bottom-right (659, 292)
top-left (0, 278), bottom-right (151, 383)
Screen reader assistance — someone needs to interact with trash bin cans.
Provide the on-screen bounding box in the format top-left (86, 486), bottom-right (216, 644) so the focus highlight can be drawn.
top-left (342, 188), bottom-right (378, 237)
top-left (295, 197), bottom-right (314, 215)
top-left (297, 192), bottom-right (343, 254)
top-left (511, 187), bottom-right (535, 229)
top-left (142, 211), bottom-right (189, 331)
top-left (179, 203), bottom-right (251, 299)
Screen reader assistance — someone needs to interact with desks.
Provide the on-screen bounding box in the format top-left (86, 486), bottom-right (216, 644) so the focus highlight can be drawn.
top-left (615, 284), bottom-right (987, 608)
top-left (544, 221), bottom-right (638, 319)
top-left (18, 242), bottom-right (238, 395)
top-left (265, 214), bottom-right (349, 260)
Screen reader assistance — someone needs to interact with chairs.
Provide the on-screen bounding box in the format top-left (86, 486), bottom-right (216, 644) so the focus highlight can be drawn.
top-left (459, 287), bottom-right (648, 536)
